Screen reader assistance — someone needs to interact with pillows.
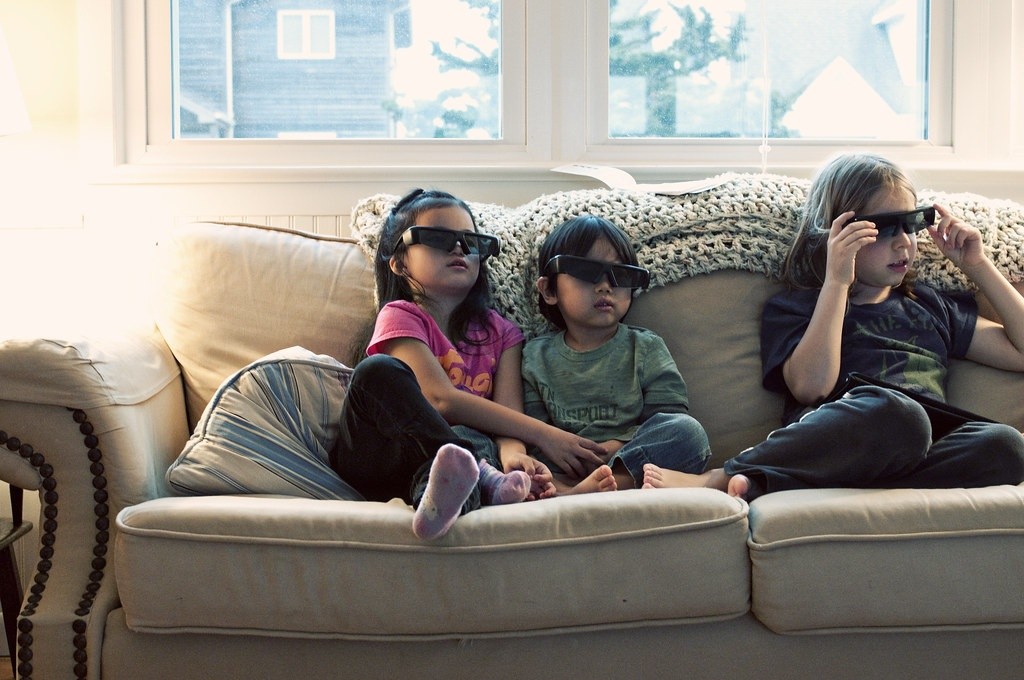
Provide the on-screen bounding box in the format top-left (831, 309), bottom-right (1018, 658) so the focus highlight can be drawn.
top-left (164, 347), bottom-right (368, 501)
top-left (150, 222), bottom-right (384, 433)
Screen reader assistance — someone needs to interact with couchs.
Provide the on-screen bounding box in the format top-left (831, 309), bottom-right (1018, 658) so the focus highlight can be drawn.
top-left (1, 175), bottom-right (1024, 680)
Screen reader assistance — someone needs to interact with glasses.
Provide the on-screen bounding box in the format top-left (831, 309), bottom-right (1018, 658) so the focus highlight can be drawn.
top-left (541, 254), bottom-right (651, 290)
top-left (393, 225), bottom-right (501, 259)
top-left (842, 205), bottom-right (936, 239)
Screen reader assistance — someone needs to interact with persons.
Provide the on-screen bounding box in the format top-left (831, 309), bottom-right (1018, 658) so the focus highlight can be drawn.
top-left (521, 213), bottom-right (711, 495)
top-left (330, 188), bottom-right (609, 543)
top-left (642, 152), bottom-right (1024, 500)
